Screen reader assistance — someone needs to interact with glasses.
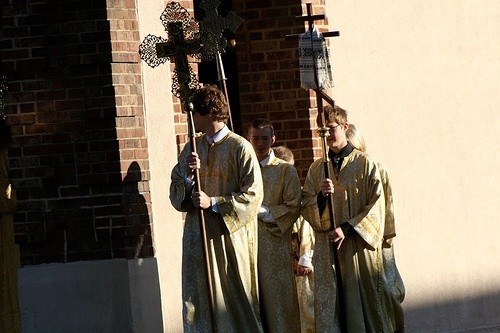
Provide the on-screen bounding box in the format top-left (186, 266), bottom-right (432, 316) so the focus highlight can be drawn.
top-left (248, 136), bottom-right (272, 143)
top-left (329, 124), bottom-right (340, 133)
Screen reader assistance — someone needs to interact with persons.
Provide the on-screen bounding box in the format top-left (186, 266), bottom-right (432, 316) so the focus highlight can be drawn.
top-left (301, 102), bottom-right (396, 332)
top-left (345, 124), bottom-right (407, 332)
top-left (169, 84), bottom-right (267, 333)
top-left (271, 146), bottom-right (296, 167)
top-left (242, 117), bottom-right (305, 333)
top-left (289, 215), bottom-right (318, 331)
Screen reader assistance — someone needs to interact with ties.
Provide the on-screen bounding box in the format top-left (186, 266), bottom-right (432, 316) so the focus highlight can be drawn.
top-left (334, 155), bottom-right (340, 181)
